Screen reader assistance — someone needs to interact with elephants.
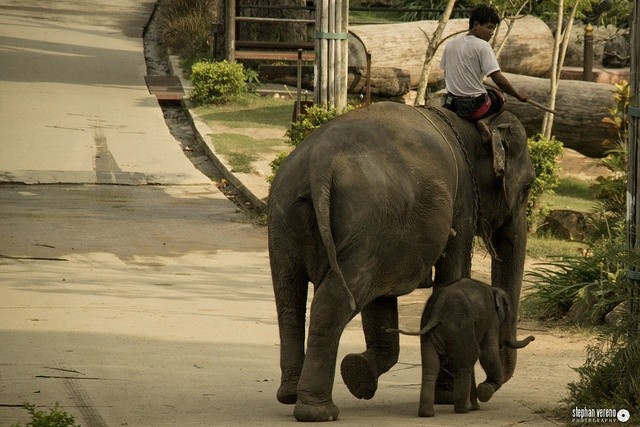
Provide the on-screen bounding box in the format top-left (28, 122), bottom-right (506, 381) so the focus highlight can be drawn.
top-left (384, 276), bottom-right (535, 418)
top-left (266, 99), bottom-right (538, 424)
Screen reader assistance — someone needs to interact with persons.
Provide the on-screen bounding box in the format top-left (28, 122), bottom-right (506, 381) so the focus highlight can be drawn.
top-left (439, 4), bottom-right (528, 119)
